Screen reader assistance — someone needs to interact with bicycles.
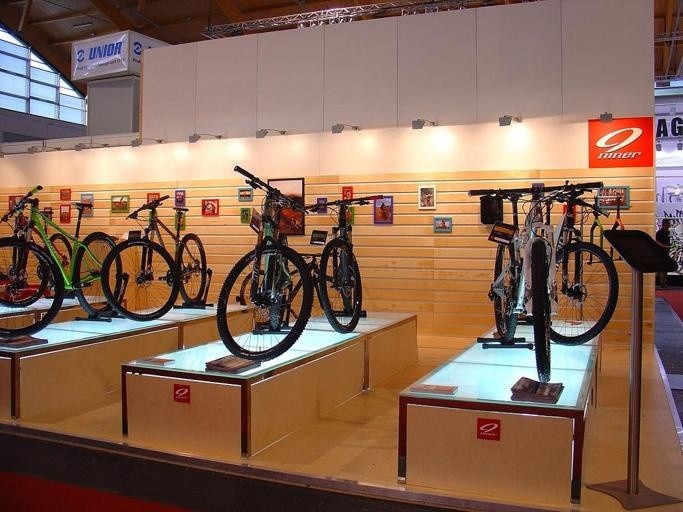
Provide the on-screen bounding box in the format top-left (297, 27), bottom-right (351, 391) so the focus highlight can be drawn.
top-left (303, 195), bottom-right (383, 333)
top-left (547, 187), bottom-right (618, 346)
top-left (0, 186), bottom-right (121, 336)
top-left (100, 195), bottom-right (206, 320)
top-left (217, 166), bottom-right (312, 361)
top-left (0, 203), bottom-right (74, 308)
top-left (468, 179), bottom-right (603, 383)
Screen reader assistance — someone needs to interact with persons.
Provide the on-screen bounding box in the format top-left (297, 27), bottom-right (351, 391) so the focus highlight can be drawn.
top-left (656, 218), bottom-right (671, 290)
